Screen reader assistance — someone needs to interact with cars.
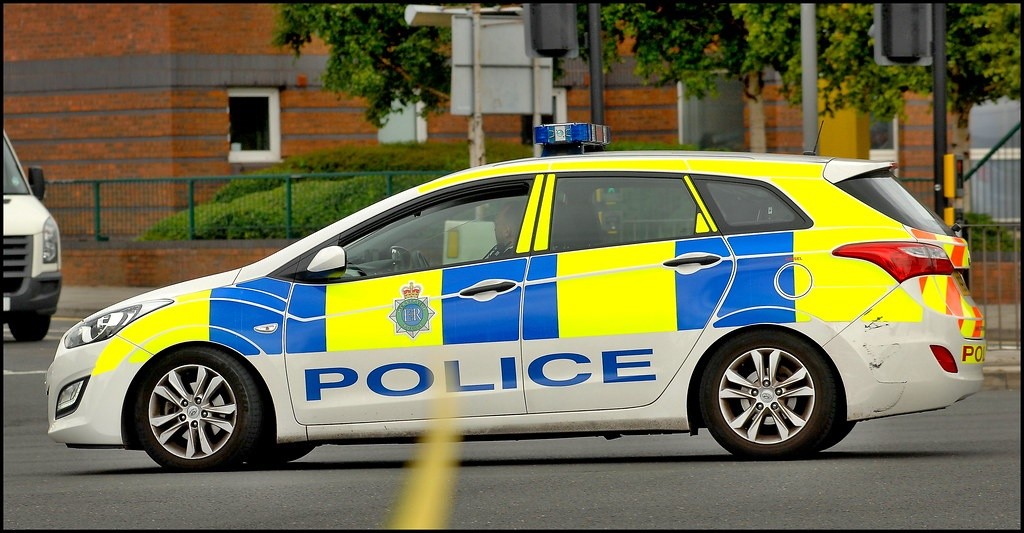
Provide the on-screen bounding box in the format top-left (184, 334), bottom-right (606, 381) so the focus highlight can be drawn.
top-left (1, 127), bottom-right (62, 342)
top-left (42, 122), bottom-right (989, 458)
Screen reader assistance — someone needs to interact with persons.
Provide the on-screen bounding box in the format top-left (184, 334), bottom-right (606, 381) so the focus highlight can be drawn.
top-left (494, 200), bottom-right (526, 258)
